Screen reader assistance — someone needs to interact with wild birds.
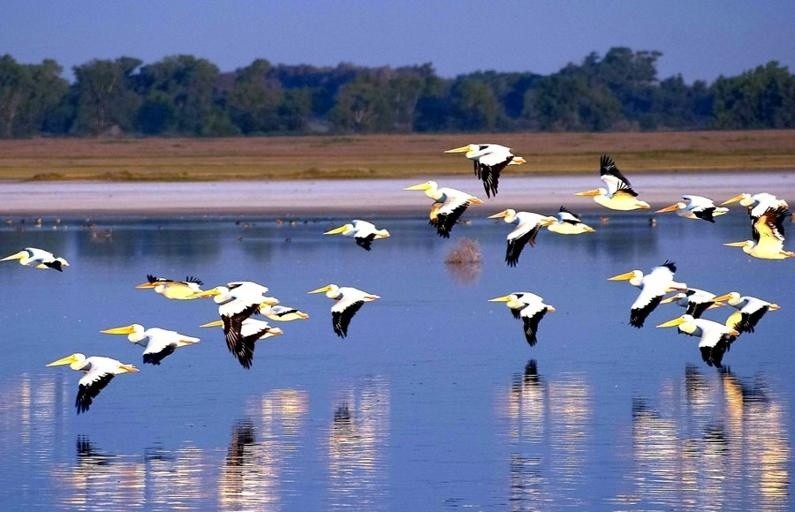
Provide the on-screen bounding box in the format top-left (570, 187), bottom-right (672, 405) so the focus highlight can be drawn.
top-left (0, 247), bottom-right (70, 272)
top-left (654, 195), bottom-right (730, 223)
top-left (718, 192), bottom-right (795, 260)
top-left (306, 283), bottom-right (381, 339)
top-left (443, 143), bottom-right (527, 199)
top-left (654, 287), bottom-right (782, 368)
top-left (99, 276), bottom-right (308, 369)
top-left (45, 354), bottom-right (141, 415)
top-left (607, 260), bottom-right (687, 328)
top-left (402, 180), bottom-right (485, 239)
top-left (486, 205), bottom-right (597, 268)
top-left (488, 292), bottom-right (556, 346)
top-left (323, 218), bottom-right (390, 251)
top-left (574, 153), bottom-right (651, 211)
